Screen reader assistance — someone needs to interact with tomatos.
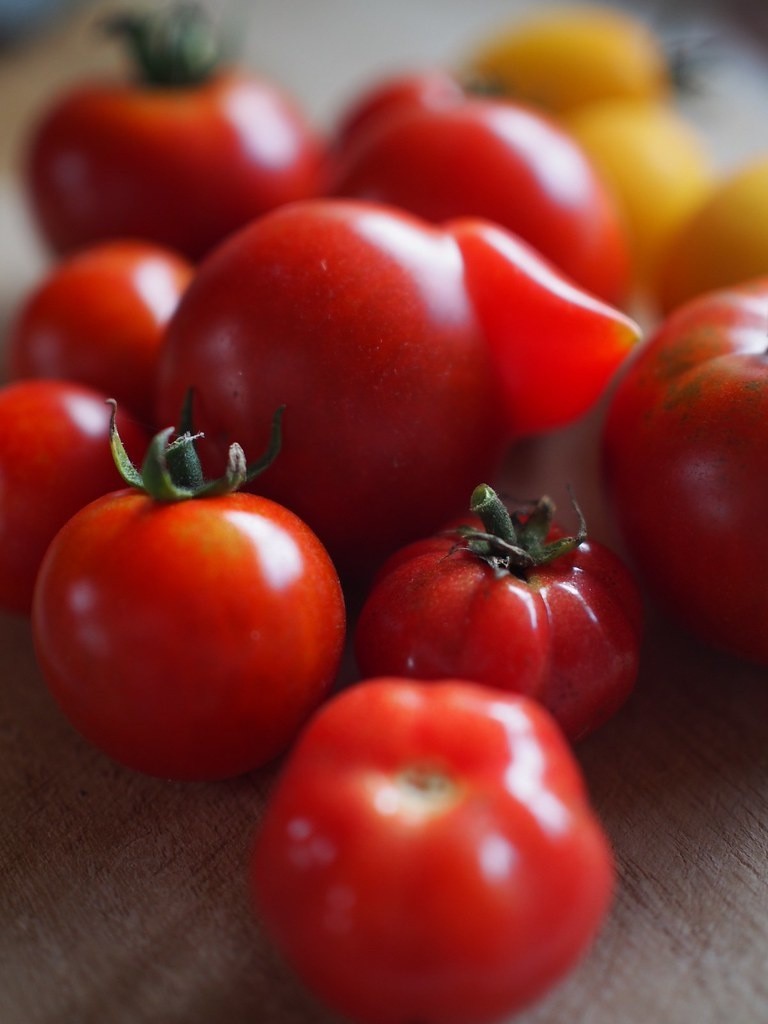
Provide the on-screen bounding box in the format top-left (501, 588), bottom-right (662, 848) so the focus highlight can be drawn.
top-left (0, 15), bottom-right (768, 1024)
